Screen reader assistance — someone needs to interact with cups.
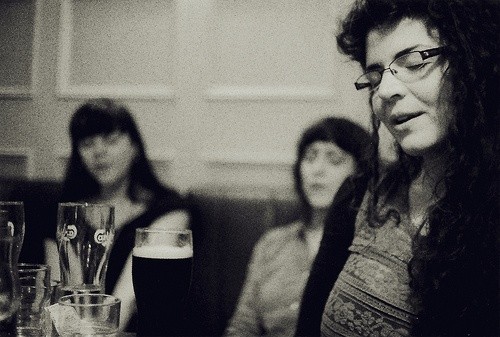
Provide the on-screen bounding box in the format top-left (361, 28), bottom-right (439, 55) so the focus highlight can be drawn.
top-left (15, 286), bottom-right (53, 337)
top-left (17, 264), bottom-right (51, 285)
top-left (0, 200), bottom-right (25, 321)
top-left (58, 201), bottom-right (115, 293)
top-left (132, 227), bottom-right (194, 337)
top-left (57, 294), bottom-right (121, 337)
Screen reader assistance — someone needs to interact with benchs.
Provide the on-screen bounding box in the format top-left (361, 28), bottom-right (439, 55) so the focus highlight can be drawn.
top-left (0, 176), bottom-right (303, 337)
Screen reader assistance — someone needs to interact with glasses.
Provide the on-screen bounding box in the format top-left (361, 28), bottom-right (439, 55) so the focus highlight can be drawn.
top-left (355, 45), bottom-right (449, 94)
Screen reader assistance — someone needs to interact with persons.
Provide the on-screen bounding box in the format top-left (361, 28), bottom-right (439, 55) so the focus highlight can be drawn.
top-left (39, 100), bottom-right (190, 337)
top-left (294, 0), bottom-right (500, 337)
top-left (226, 117), bottom-right (376, 337)
